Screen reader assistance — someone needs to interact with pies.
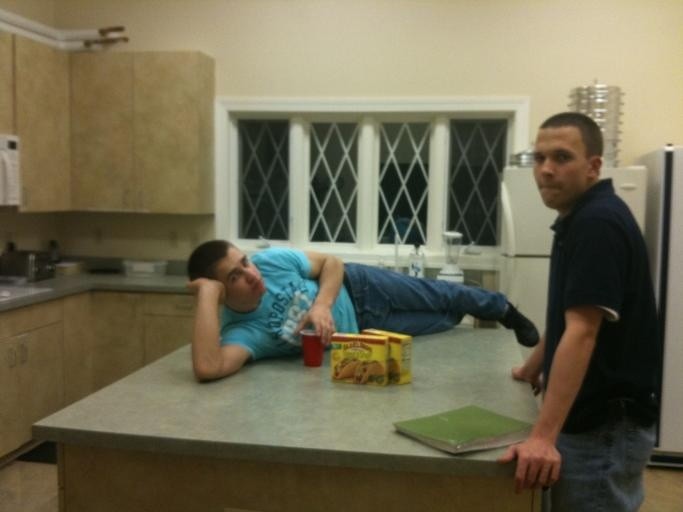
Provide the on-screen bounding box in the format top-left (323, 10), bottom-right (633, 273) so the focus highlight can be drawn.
top-left (333, 357), bottom-right (361, 379)
top-left (354, 360), bottom-right (385, 385)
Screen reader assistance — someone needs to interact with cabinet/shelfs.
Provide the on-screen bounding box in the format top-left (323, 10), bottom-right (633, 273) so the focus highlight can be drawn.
top-left (141, 289), bottom-right (226, 369)
top-left (61, 285), bottom-right (91, 407)
top-left (0, 298), bottom-right (65, 474)
top-left (70, 46), bottom-right (216, 221)
top-left (0, 28), bottom-right (71, 216)
top-left (85, 286), bottom-right (143, 396)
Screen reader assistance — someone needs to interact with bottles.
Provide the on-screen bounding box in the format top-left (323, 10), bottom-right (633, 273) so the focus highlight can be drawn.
top-left (408, 241), bottom-right (426, 278)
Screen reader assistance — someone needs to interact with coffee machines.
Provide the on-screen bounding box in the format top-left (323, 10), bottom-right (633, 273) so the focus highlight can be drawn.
top-left (436, 231), bottom-right (464, 283)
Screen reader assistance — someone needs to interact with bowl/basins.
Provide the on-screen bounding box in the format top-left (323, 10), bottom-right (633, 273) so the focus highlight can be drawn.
top-left (121, 259), bottom-right (168, 278)
top-left (55, 261), bottom-right (86, 275)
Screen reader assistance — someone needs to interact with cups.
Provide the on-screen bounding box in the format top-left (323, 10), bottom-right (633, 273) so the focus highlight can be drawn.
top-left (299, 329), bottom-right (325, 368)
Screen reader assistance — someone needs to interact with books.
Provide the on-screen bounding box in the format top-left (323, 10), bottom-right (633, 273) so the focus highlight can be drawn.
top-left (390, 403), bottom-right (536, 457)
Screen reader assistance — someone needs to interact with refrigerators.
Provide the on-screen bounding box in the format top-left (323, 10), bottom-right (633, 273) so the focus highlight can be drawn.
top-left (500, 166), bottom-right (647, 409)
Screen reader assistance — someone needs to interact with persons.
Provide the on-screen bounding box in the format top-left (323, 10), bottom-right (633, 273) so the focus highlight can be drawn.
top-left (186, 240), bottom-right (540, 385)
top-left (496, 113), bottom-right (661, 512)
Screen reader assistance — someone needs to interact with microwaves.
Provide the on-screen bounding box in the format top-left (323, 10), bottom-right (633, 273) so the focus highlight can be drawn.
top-left (0, 133), bottom-right (21, 206)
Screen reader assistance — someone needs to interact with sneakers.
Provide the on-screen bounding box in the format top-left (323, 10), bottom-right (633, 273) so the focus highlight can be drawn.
top-left (499, 295), bottom-right (539, 349)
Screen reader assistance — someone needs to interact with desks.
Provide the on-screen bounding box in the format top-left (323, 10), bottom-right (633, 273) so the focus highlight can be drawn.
top-left (28, 324), bottom-right (547, 512)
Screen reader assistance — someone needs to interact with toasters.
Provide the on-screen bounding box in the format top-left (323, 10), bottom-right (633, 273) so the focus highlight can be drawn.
top-left (1, 249), bottom-right (55, 282)
